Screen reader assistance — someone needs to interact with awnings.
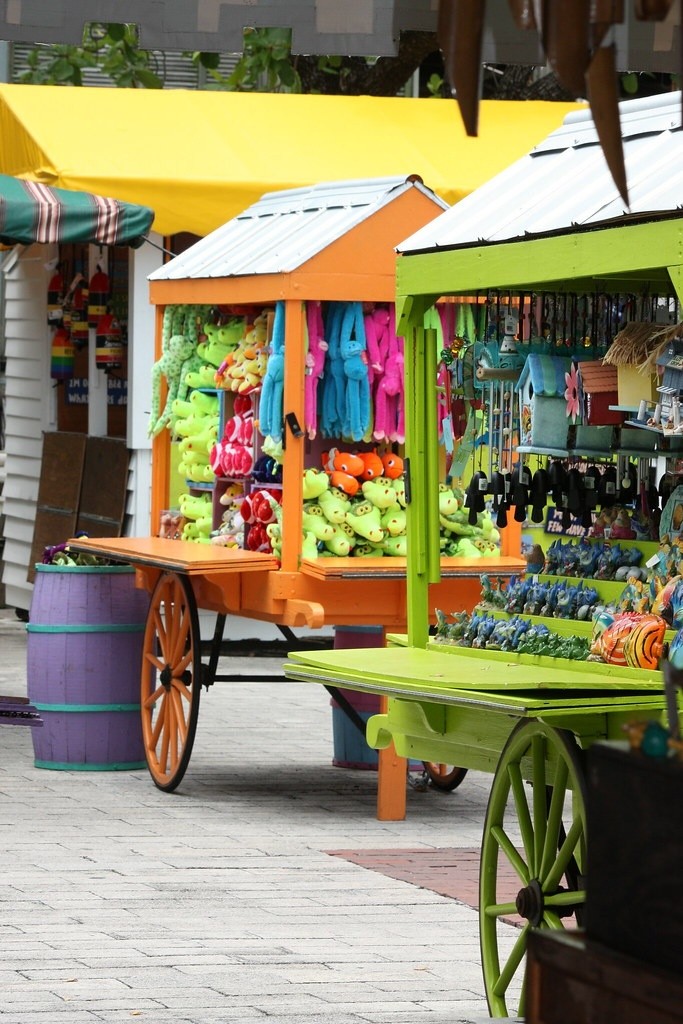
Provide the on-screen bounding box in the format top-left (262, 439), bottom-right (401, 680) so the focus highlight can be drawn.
top-left (0, 171), bottom-right (154, 248)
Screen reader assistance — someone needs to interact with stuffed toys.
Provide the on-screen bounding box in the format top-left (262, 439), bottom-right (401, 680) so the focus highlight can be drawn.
top-left (146, 298), bottom-right (510, 563)
